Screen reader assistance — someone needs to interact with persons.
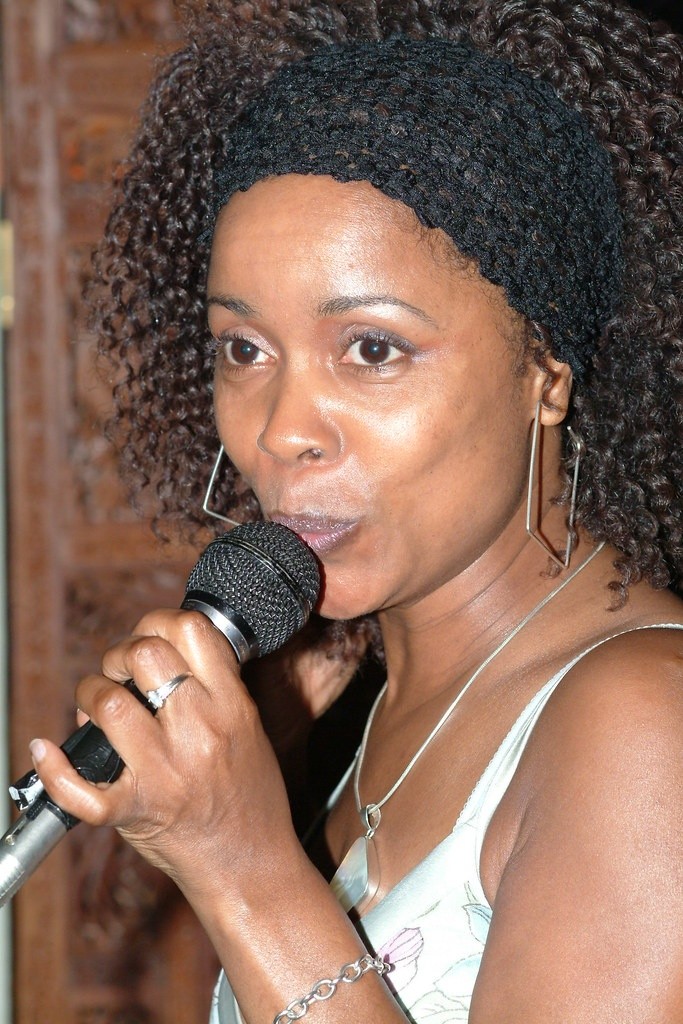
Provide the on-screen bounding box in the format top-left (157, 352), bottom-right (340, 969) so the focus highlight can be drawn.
top-left (27, 0), bottom-right (683, 1024)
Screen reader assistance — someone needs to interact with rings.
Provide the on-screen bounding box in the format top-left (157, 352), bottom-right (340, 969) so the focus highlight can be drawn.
top-left (146, 671), bottom-right (193, 710)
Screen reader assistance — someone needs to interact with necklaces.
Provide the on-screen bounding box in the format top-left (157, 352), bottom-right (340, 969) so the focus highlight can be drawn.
top-left (330, 539), bottom-right (608, 919)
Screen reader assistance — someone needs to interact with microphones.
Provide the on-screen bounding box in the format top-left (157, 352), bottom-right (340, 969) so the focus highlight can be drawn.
top-left (0, 520), bottom-right (322, 910)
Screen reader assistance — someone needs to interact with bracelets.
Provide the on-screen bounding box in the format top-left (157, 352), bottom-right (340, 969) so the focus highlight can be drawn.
top-left (273, 953), bottom-right (391, 1024)
